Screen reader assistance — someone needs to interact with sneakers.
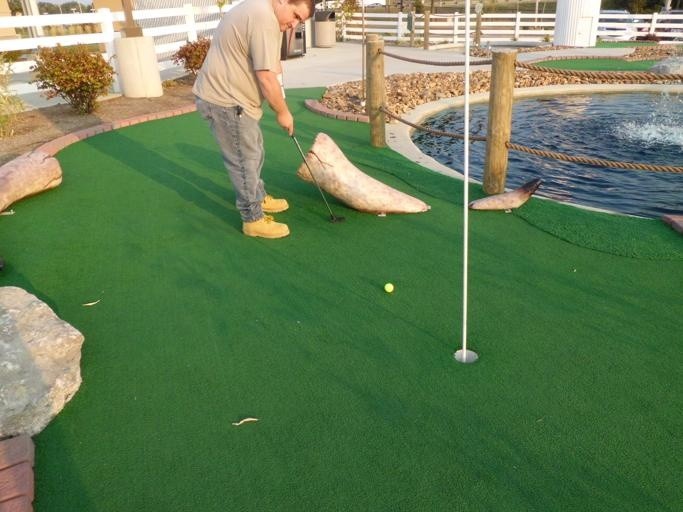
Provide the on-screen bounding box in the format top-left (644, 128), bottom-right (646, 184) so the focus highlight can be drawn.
top-left (260, 194), bottom-right (290, 214)
top-left (242, 213), bottom-right (290, 239)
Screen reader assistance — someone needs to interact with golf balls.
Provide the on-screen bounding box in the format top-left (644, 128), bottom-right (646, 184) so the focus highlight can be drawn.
top-left (384, 283), bottom-right (394, 293)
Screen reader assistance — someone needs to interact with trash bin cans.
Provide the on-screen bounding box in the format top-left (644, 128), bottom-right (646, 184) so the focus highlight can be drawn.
top-left (314, 11), bottom-right (336, 48)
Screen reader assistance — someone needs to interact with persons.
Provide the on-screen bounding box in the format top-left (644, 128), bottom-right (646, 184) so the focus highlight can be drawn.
top-left (191, 0), bottom-right (319, 239)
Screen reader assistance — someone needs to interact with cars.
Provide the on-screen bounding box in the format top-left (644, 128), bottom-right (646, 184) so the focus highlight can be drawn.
top-left (598, 9), bottom-right (683, 41)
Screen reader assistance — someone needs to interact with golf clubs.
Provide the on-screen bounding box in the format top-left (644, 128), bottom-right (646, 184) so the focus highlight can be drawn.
top-left (292, 135), bottom-right (346, 223)
